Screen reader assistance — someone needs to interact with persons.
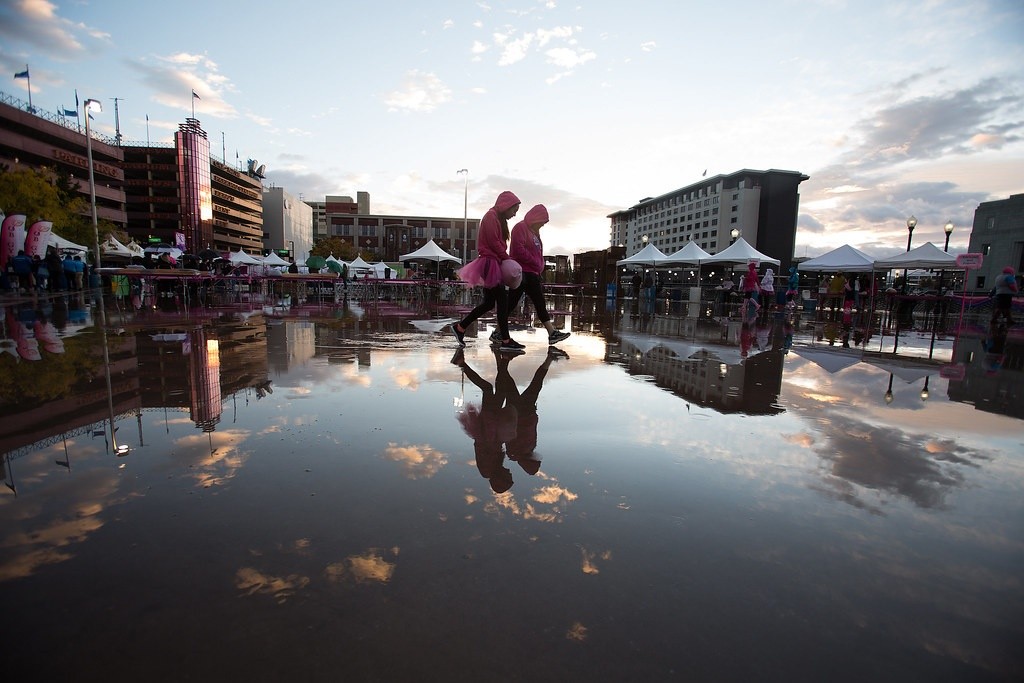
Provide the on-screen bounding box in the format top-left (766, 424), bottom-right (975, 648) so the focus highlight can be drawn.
top-left (131, 278), bottom-right (157, 310)
top-left (288, 261), bottom-right (298, 273)
top-left (343, 263), bottom-right (348, 282)
top-left (451, 191), bottom-right (571, 350)
top-left (5, 246), bottom-right (116, 294)
top-left (450, 345), bottom-right (570, 494)
top-left (142, 251), bottom-right (240, 275)
top-left (988, 266), bottom-right (1018, 328)
top-left (19, 297), bottom-right (88, 332)
top-left (722, 263), bottom-right (897, 311)
top-left (631, 271), bottom-right (653, 298)
top-left (739, 312), bottom-right (873, 356)
top-left (308, 268), bottom-right (321, 274)
top-left (982, 328), bottom-right (1009, 377)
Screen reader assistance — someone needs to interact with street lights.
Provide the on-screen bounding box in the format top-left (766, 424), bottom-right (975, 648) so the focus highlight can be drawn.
top-left (885, 301), bottom-right (902, 404)
top-left (726, 227), bottom-right (738, 318)
top-left (457, 169), bottom-right (468, 265)
top-left (920, 302), bottom-right (943, 401)
top-left (641, 234), bottom-right (650, 287)
top-left (938, 220), bottom-right (954, 295)
top-left (83, 96), bottom-right (103, 289)
top-left (97, 286), bottom-right (130, 457)
top-left (900, 215), bottom-right (916, 296)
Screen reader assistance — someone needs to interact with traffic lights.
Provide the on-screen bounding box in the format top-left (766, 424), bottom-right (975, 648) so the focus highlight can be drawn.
top-left (264, 248), bottom-right (269, 257)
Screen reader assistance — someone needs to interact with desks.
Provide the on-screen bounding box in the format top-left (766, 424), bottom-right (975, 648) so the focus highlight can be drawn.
top-left (660, 288), bottom-right (682, 302)
top-left (545, 283), bottom-right (586, 299)
top-left (350, 276), bottom-right (470, 306)
top-left (706, 289), bottom-right (742, 304)
top-left (95, 268), bottom-right (341, 303)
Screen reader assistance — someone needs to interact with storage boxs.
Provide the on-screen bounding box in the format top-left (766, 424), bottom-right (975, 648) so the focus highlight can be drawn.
top-left (801, 289), bottom-right (818, 310)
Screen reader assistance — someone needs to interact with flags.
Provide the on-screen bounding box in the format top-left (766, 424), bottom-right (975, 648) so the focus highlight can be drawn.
top-left (193, 91), bottom-right (200, 99)
top-left (14, 71), bottom-right (77, 117)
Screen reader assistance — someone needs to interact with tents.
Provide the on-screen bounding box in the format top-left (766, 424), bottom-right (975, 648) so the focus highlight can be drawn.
top-left (798, 242), bottom-right (957, 297)
top-left (399, 240), bottom-right (462, 280)
top-left (228, 249), bottom-right (397, 279)
top-left (617, 327), bottom-right (756, 364)
top-left (790, 328), bottom-right (947, 385)
top-left (616, 236), bottom-right (781, 299)
top-left (46, 230), bottom-right (145, 265)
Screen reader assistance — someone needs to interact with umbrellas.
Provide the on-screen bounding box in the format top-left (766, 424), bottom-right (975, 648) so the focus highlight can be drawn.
top-left (145, 242), bottom-right (231, 264)
top-left (305, 256), bottom-right (343, 273)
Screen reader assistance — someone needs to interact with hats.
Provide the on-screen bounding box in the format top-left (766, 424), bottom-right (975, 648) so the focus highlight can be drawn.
top-left (292, 260), bottom-right (296, 264)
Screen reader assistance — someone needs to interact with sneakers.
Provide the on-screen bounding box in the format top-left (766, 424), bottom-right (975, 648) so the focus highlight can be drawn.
top-left (549, 331), bottom-right (571, 345)
top-left (498, 338), bottom-right (526, 350)
top-left (489, 330), bottom-right (502, 343)
top-left (548, 346), bottom-right (570, 361)
top-left (451, 323), bottom-right (466, 347)
top-left (499, 347), bottom-right (526, 360)
top-left (489, 344), bottom-right (499, 353)
top-left (449, 346), bottom-right (464, 365)
